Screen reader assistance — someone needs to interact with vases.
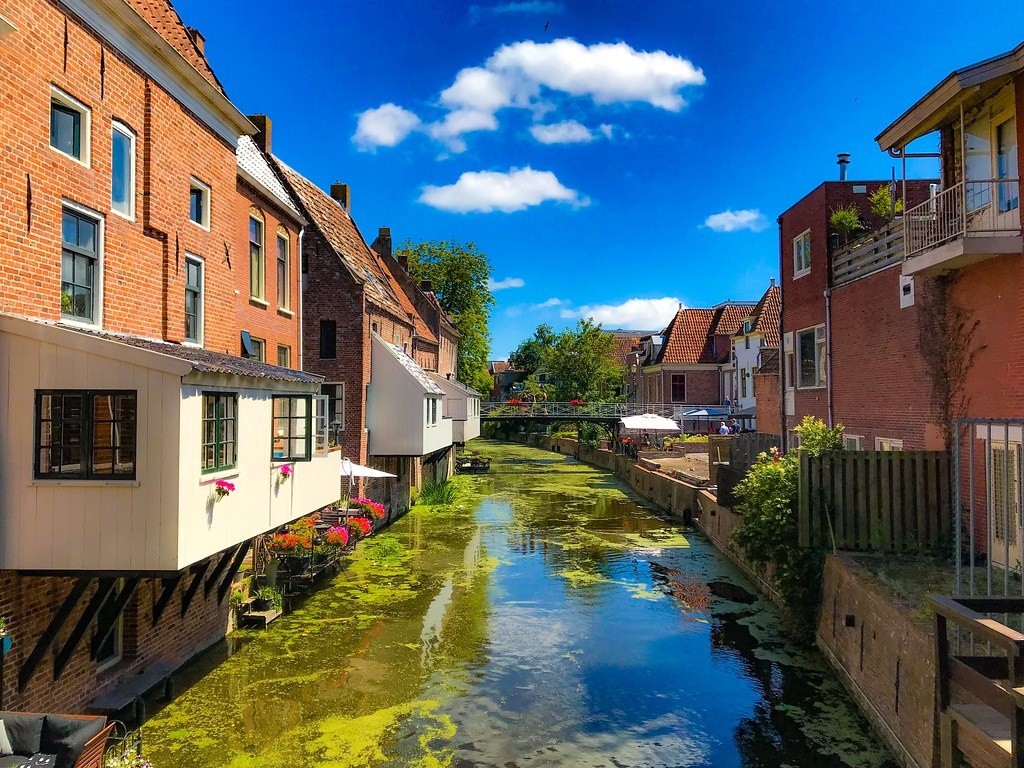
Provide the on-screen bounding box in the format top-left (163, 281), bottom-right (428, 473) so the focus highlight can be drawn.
top-left (314, 554), bottom-right (329, 563)
top-left (286, 556), bottom-right (311, 574)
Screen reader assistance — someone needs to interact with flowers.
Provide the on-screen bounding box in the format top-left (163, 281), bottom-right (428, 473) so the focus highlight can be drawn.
top-left (266, 496), bottom-right (386, 557)
top-left (280, 464), bottom-right (293, 485)
top-left (508, 399), bottom-right (520, 404)
top-left (214, 479), bottom-right (235, 503)
top-left (570, 400), bottom-right (585, 408)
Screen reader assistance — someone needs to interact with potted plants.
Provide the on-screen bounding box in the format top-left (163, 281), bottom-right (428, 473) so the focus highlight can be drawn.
top-left (252, 585), bottom-right (282, 612)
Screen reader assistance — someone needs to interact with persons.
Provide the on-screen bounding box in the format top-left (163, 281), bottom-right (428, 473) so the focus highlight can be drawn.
top-left (719, 418), bottom-right (741, 435)
top-left (618, 433), bottom-right (638, 461)
top-left (723, 396), bottom-right (732, 405)
top-left (643, 429), bottom-right (649, 442)
top-left (521, 389), bottom-right (548, 416)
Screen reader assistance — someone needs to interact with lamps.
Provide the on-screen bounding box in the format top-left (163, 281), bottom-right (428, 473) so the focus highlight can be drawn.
top-left (329, 418), bottom-right (341, 446)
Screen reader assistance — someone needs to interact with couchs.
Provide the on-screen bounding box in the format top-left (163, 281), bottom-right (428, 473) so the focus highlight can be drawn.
top-left (0, 711), bottom-right (116, 768)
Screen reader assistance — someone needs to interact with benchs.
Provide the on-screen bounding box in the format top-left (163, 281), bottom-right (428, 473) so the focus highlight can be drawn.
top-left (320, 507), bottom-right (361, 525)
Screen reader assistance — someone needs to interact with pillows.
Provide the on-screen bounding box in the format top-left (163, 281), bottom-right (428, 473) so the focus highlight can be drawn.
top-left (0, 718), bottom-right (14, 756)
top-left (53, 717), bottom-right (105, 768)
top-left (0, 712), bottom-right (45, 756)
top-left (40, 714), bottom-right (91, 753)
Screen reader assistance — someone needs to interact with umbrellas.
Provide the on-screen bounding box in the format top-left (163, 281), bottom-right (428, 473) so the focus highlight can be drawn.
top-left (681, 407), bottom-right (728, 434)
top-left (341, 459), bottom-right (398, 478)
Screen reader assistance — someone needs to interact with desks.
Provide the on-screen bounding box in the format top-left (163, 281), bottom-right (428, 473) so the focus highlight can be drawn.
top-left (314, 524), bottom-right (331, 529)
